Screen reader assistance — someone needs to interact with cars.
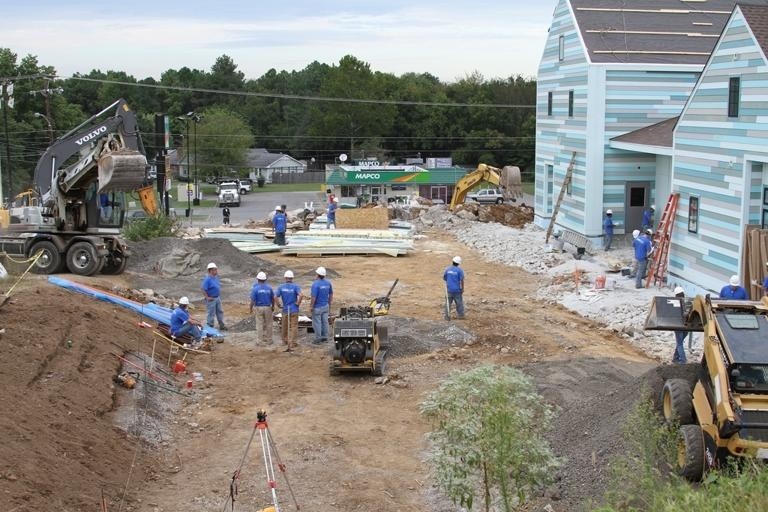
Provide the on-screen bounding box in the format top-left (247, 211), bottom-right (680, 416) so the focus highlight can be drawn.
top-left (147, 170), bottom-right (159, 180)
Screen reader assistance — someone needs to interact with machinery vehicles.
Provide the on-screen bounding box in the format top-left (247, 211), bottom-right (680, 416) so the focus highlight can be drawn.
top-left (329, 278), bottom-right (400, 377)
top-left (1, 97), bottom-right (162, 277)
top-left (641, 292), bottom-right (766, 482)
top-left (450, 162), bottom-right (524, 213)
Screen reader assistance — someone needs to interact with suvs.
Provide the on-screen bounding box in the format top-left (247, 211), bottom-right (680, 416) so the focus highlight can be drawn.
top-left (468, 188), bottom-right (507, 204)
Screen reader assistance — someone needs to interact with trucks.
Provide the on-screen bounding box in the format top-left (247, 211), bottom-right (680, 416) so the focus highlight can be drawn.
top-left (207, 176), bottom-right (253, 208)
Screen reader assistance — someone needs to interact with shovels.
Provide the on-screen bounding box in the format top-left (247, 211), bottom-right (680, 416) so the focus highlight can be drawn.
top-left (443, 280), bottom-right (454, 320)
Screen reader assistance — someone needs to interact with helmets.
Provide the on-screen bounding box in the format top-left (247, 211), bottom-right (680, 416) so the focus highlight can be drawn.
top-left (333, 197), bottom-right (339, 202)
top-left (606, 204), bottom-right (741, 297)
top-left (178, 256), bottom-right (462, 305)
top-left (275, 206), bottom-right (281, 211)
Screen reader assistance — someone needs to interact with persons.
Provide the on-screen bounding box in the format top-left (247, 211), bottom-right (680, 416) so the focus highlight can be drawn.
top-left (603, 209), bottom-right (621, 251)
top-left (631, 204), bottom-right (656, 290)
top-left (308, 265), bottom-right (334, 345)
top-left (249, 271), bottom-right (276, 346)
top-left (170, 296), bottom-right (205, 345)
top-left (222, 203), bottom-right (291, 246)
top-left (750, 262), bottom-right (768, 296)
top-left (273, 270), bottom-right (305, 348)
top-left (442, 255), bottom-right (466, 320)
top-left (671, 286), bottom-right (688, 363)
top-left (719, 274), bottom-right (750, 300)
top-left (325, 195), bottom-right (338, 229)
top-left (199, 262), bottom-right (229, 331)
top-left (90, 190), bottom-right (113, 223)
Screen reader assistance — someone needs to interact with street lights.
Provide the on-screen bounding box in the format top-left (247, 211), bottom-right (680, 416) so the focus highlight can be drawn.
top-left (191, 115), bottom-right (202, 206)
top-left (0, 77), bottom-right (17, 208)
top-left (177, 111), bottom-right (192, 216)
top-left (33, 111), bottom-right (54, 144)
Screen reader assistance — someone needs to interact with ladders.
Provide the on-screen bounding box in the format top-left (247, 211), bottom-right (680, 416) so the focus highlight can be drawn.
top-left (645, 193), bottom-right (680, 288)
top-left (545, 152), bottom-right (576, 244)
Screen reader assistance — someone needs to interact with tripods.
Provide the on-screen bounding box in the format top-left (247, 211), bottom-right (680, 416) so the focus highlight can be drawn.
top-left (222, 409), bottom-right (301, 512)
